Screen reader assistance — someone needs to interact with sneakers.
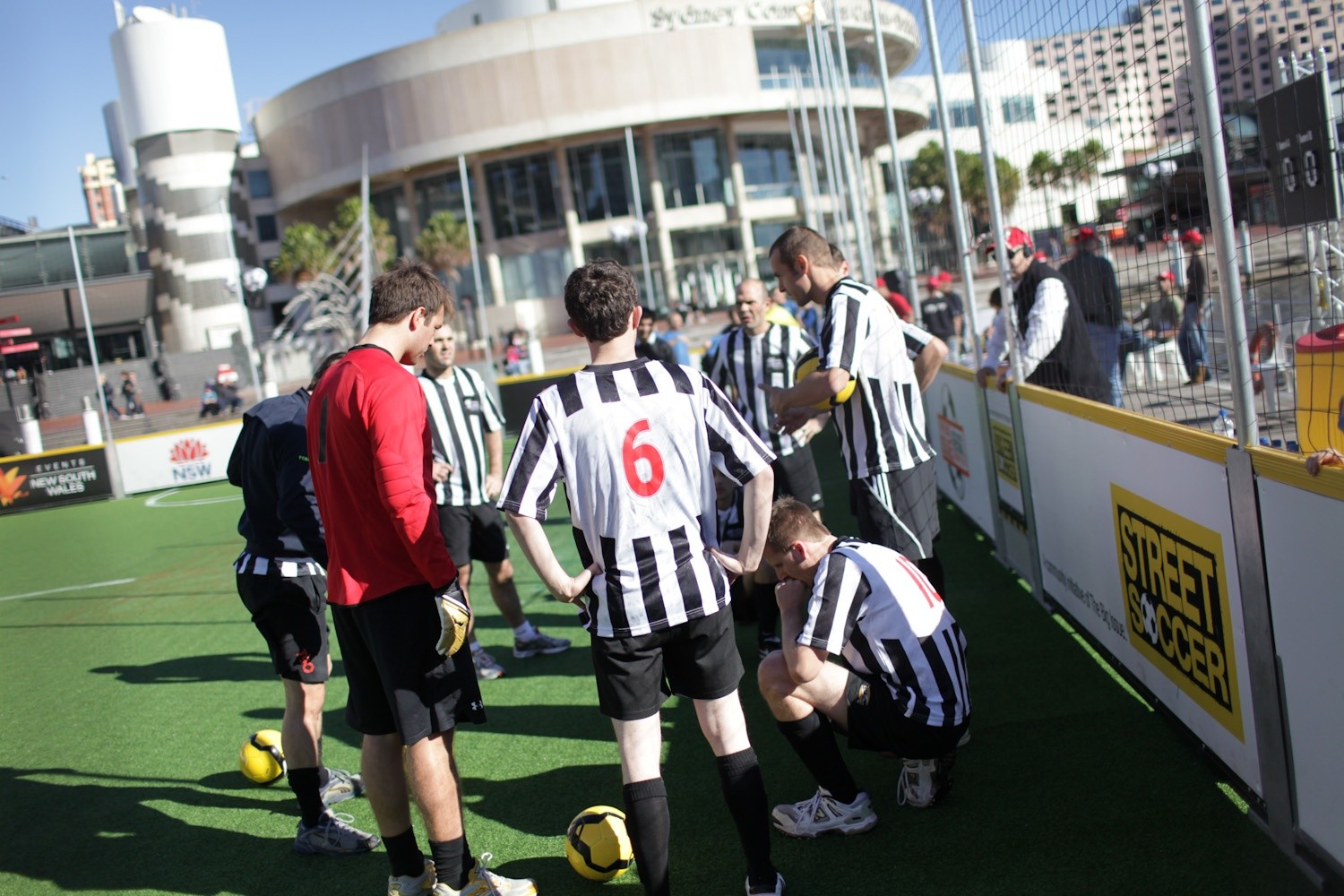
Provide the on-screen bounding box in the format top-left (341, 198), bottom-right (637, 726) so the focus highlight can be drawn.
top-left (771, 784), bottom-right (878, 836)
top-left (385, 850), bottom-right (436, 896)
top-left (514, 625), bottom-right (573, 657)
top-left (296, 809), bottom-right (383, 856)
top-left (472, 649), bottom-right (506, 680)
top-left (319, 767), bottom-right (366, 806)
top-left (896, 755), bottom-right (963, 807)
top-left (433, 851), bottom-right (537, 896)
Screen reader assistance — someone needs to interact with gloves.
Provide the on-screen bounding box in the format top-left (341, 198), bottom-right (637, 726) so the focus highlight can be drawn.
top-left (433, 580), bottom-right (473, 659)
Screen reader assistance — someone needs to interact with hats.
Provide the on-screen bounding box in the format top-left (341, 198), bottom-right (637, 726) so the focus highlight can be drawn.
top-left (1179, 230), bottom-right (1202, 243)
top-left (986, 227), bottom-right (1033, 256)
top-left (1075, 229), bottom-right (1100, 244)
top-left (1157, 272), bottom-right (1174, 280)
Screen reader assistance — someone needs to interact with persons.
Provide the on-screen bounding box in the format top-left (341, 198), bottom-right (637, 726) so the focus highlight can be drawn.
top-left (976, 227), bottom-right (1113, 407)
top-left (634, 307), bottom-right (690, 367)
top-left (227, 352), bottom-right (380, 851)
top-left (1120, 271), bottom-right (1184, 380)
top-left (876, 272), bottom-right (966, 364)
top-left (1057, 226), bottom-right (1123, 407)
top-left (308, 263), bottom-right (537, 896)
top-left (722, 286), bottom-right (819, 344)
top-left (701, 278), bottom-right (831, 662)
top-left (828, 242), bottom-right (948, 392)
top-left (5, 366), bottom-right (27, 388)
top-left (712, 465), bottom-right (757, 623)
top-left (417, 322), bottom-right (571, 680)
top-left (502, 331), bottom-right (529, 377)
top-left (199, 379), bottom-right (242, 418)
top-left (1039, 238), bottom-right (1061, 265)
top-left (95, 371), bottom-right (144, 420)
top-left (1176, 229), bottom-right (1212, 386)
top-left (496, 258), bottom-right (797, 896)
top-left (758, 496), bottom-right (974, 839)
top-left (757, 226), bottom-right (971, 746)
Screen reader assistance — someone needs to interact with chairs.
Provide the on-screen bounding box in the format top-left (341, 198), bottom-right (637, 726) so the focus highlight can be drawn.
top-left (1123, 330), bottom-right (1191, 390)
top-left (1249, 314), bottom-right (1311, 420)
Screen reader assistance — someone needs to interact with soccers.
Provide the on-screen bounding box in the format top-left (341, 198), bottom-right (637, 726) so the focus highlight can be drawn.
top-left (793, 346), bottom-right (858, 411)
top-left (565, 804), bottom-right (633, 882)
top-left (240, 730), bottom-right (288, 786)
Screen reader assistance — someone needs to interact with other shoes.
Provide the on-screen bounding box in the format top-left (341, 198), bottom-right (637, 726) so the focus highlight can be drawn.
top-left (757, 638), bottom-right (783, 660)
top-left (1196, 364), bottom-right (1208, 385)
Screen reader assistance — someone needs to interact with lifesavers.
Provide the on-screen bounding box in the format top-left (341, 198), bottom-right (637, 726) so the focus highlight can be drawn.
top-left (1249, 321), bottom-right (1274, 394)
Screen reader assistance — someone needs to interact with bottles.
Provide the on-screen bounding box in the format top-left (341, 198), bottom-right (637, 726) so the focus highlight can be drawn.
top-left (1260, 437), bottom-right (1268, 444)
top-left (1213, 408), bottom-right (1234, 439)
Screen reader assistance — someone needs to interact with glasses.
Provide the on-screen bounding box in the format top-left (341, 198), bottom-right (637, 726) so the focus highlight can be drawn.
top-left (992, 248), bottom-right (1024, 262)
